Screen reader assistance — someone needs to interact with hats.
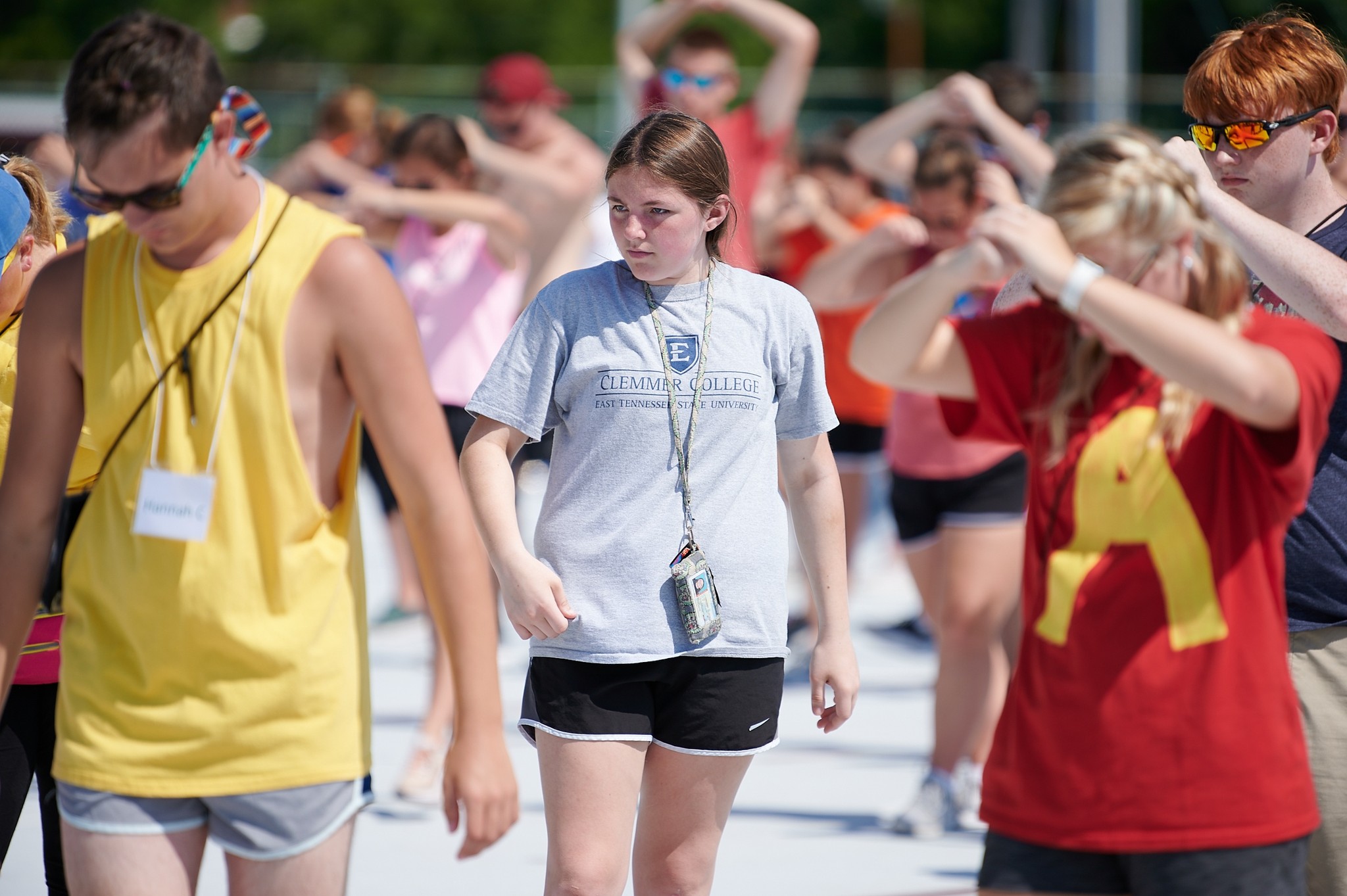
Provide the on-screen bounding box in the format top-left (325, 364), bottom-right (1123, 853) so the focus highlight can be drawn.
top-left (481, 53), bottom-right (571, 106)
top-left (1, 169), bottom-right (33, 277)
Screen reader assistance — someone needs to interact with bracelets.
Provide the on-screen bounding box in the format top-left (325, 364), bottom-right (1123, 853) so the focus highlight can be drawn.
top-left (1059, 251), bottom-right (1103, 314)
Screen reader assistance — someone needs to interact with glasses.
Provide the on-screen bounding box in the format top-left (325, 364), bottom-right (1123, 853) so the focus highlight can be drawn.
top-left (918, 169), bottom-right (959, 187)
top-left (1187, 104), bottom-right (1335, 154)
top-left (67, 123), bottom-right (215, 212)
top-left (662, 68), bottom-right (711, 91)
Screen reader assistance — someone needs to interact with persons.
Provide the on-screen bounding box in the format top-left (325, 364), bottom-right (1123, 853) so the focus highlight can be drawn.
top-left (0, 0), bottom-right (1346, 896)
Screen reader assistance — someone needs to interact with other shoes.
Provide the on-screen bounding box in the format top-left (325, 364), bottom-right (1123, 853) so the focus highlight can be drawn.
top-left (894, 755), bottom-right (991, 835)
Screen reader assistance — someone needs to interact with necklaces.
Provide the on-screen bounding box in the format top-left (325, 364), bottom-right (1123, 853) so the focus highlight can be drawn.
top-left (1251, 201), bottom-right (1346, 298)
